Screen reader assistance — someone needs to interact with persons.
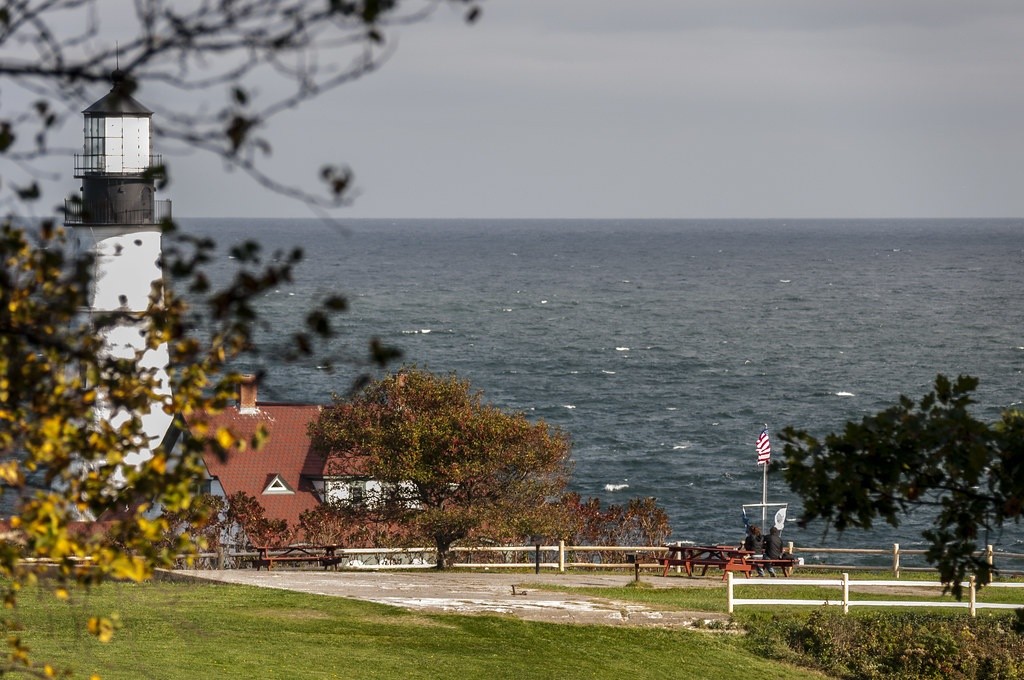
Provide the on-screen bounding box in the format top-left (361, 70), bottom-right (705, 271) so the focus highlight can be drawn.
top-left (763, 527), bottom-right (784, 578)
top-left (745, 525), bottom-right (765, 576)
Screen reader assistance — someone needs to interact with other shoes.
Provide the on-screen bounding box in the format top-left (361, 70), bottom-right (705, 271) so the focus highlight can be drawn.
top-left (756, 574), bottom-right (765, 577)
top-left (771, 573), bottom-right (776, 577)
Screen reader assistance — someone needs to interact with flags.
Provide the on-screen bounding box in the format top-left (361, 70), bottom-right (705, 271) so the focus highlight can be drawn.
top-left (756, 428), bottom-right (771, 466)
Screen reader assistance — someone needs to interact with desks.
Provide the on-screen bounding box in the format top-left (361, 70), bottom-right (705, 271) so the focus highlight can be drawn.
top-left (256, 543), bottom-right (342, 572)
top-left (656, 547), bottom-right (757, 581)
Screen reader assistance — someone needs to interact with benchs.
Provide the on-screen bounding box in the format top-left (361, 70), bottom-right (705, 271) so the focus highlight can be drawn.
top-left (652, 557), bottom-right (797, 565)
top-left (244, 556), bottom-right (349, 562)
